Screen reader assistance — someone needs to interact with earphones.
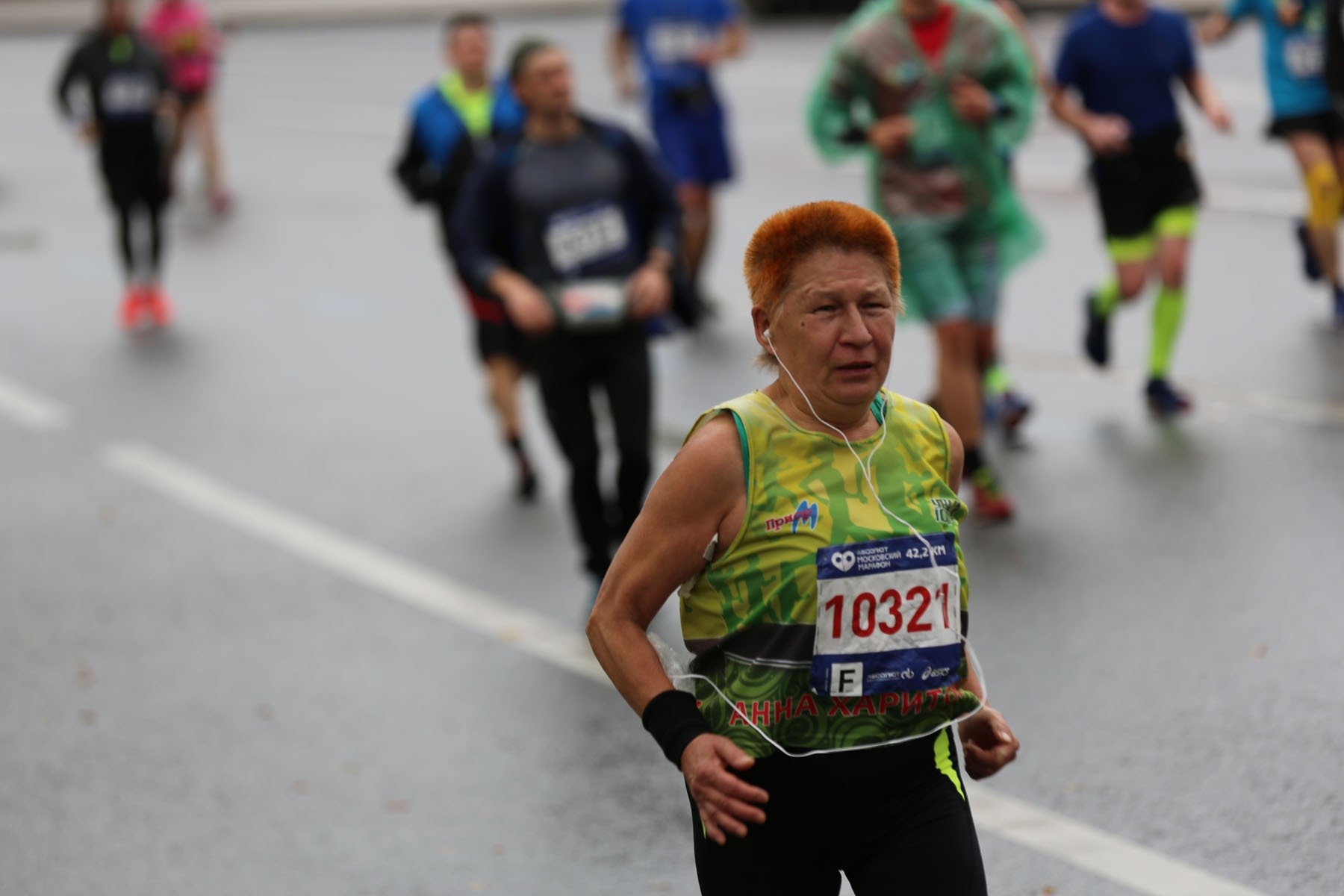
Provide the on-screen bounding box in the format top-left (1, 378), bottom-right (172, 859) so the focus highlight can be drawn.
top-left (763, 328), bottom-right (770, 339)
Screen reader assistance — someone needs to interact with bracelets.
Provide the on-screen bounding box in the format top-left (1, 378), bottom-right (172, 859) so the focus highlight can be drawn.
top-left (643, 687), bottom-right (712, 773)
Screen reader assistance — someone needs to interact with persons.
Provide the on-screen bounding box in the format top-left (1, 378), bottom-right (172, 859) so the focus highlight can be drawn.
top-left (599, 1), bottom-right (749, 332)
top-left (51, 0), bottom-right (203, 331)
top-left (443, 38), bottom-right (684, 586)
top-left (586, 200), bottom-right (1019, 896)
top-left (141, 0), bottom-right (236, 219)
top-left (394, 10), bottom-right (540, 500)
top-left (806, 1), bottom-right (1344, 523)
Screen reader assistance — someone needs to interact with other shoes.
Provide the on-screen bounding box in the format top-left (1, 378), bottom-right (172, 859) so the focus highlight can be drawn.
top-left (1333, 283), bottom-right (1344, 315)
top-left (503, 432), bottom-right (545, 501)
top-left (120, 283), bottom-right (174, 330)
top-left (1295, 223), bottom-right (1328, 279)
top-left (1084, 294), bottom-right (1110, 365)
top-left (212, 197), bottom-right (236, 219)
top-left (1146, 378), bottom-right (1190, 418)
top-left (973, 473), bottom-right (1015, 520)
top-left (986, 382), bottom-right (1034, 433)
top-left (675, 281), bottom-right (715, 325)
